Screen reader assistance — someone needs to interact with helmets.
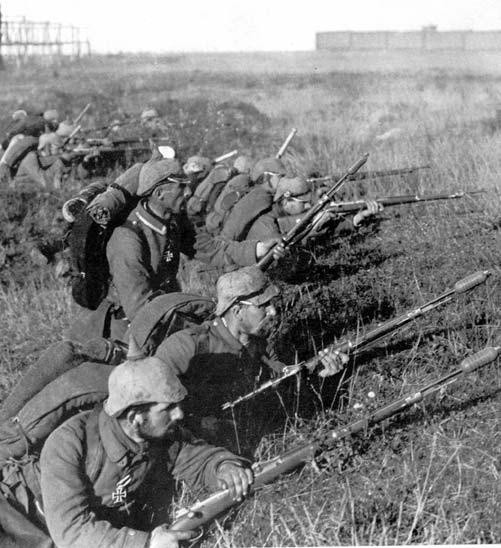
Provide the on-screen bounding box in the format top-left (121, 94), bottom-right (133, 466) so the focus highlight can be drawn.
top-left (37, 132), bottom-right (63, 150)
top-left (136, 159), bottom-right (190, 194)
top-left (215, 254), bottom-right (280, 316)
top-left (232, 154), bottom-right (312, 201)
top-left (103, 335), bottom-right (187, 418)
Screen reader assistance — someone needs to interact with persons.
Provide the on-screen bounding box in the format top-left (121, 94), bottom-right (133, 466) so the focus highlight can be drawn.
top-left (153, 265), bottom-right (349, 444)
top-left (68, 157), bottom-right (291, 340)
top-left (1, 109), bottom-right (76, 192)
top-left (12, 357), bottom-right (254, 548)
top-left (189, 155), bottom-right (385, 272)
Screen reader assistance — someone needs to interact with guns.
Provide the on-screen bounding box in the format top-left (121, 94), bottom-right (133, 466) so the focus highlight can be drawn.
top-left (324, 188), bottom-right (490, 213)
top-left (308, 164), bottom-right (430, 186)
top-left (257, 152), bottom-right (368, 272)
top-left (81, 116), bottom-right (145, 138)
top-left (214, 269), bottom-right (490, 417)
top-left (58, 138), bottom-right (173, 160)
top-left (168, 340), bottom-right (501, 546)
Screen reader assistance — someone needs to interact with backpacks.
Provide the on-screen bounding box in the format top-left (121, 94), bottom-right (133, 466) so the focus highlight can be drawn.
top-left (0, 136), bottom-right (39, 183)
top-left (71, 189), bottom-right (145, 312)
top-left (222, 186), bottom-right (274, 242)
top-left (130, 293), bottom-right (214, 358)
